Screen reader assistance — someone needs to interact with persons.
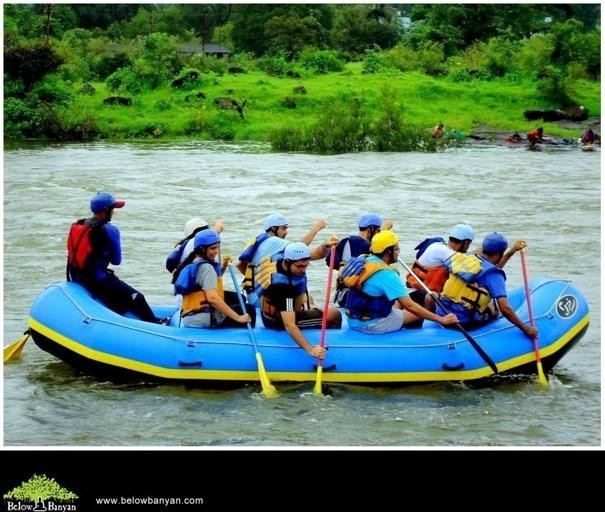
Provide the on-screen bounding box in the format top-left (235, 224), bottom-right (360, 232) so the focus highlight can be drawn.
top-left (237, 212), bottom-right (327, 307)
top-left (527, 126), bottom-right (547, 150)
top-left (165, 215), bottom-right (223, 272)
top-left (325, 213), bottom-right (394, 308)
top-left (64, 192), bottom-right (170, 334)
top-left (406, 224), bottom-right (484, 314)
top-left (344, 230), bottom-right (460, 335)
top-left (581, 127), bottom-right (597, 146)
top-left (431, 122), bottom-right (445, 139)
top-left (176, 229), bottom-right (257, 330)
top-left (504, 133), bottom-right (523, 144)
top-left (260, 234), bottom-right (342, 360)
top-left (436, 232), bottom-right (538, 339)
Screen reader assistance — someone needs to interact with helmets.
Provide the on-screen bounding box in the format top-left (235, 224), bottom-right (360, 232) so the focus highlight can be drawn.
top-left (481, 231), bottom-right (508, 252)
top-left (183, 217), bottom-right (208, 238)
top-left (357, 213), bottom-right (382, 228)
top-left (263, 213), bottom-right (289, 231)
top-left (371, 230), bottom-right (399, 255)
top-left (283, 241), bottom-right (312, 264)
top-left (448, 223), bottom-right (475, 241)
top-left (193, 228), bottom-right (222, 252)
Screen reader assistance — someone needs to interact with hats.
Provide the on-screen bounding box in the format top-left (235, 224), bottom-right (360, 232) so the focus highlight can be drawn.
top-left (90, 190), bottom-right (125, 212)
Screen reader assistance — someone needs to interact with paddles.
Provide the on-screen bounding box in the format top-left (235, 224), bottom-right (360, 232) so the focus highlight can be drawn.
top-left (223, 257), bottom-right (279, 398)
top-left (519, 243), bottom-right (548, 385)
top-left (397, 257), bottom-right (499, 375)
top-left (4, 334), bottom-right (31, 363)
top-left (313, 238), bottom-right (337, 394)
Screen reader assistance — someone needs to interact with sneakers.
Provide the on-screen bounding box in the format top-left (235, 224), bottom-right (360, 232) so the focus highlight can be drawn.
top-left (159, 316), bottom-right (171, 325)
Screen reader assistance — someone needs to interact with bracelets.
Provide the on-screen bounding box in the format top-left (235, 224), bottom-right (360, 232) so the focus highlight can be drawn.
top-left (324, 242), bottom-right (330, 250)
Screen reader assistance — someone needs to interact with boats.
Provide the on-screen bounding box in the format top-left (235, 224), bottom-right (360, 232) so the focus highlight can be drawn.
top-left (19, 271), bottom-right (593, 395)
